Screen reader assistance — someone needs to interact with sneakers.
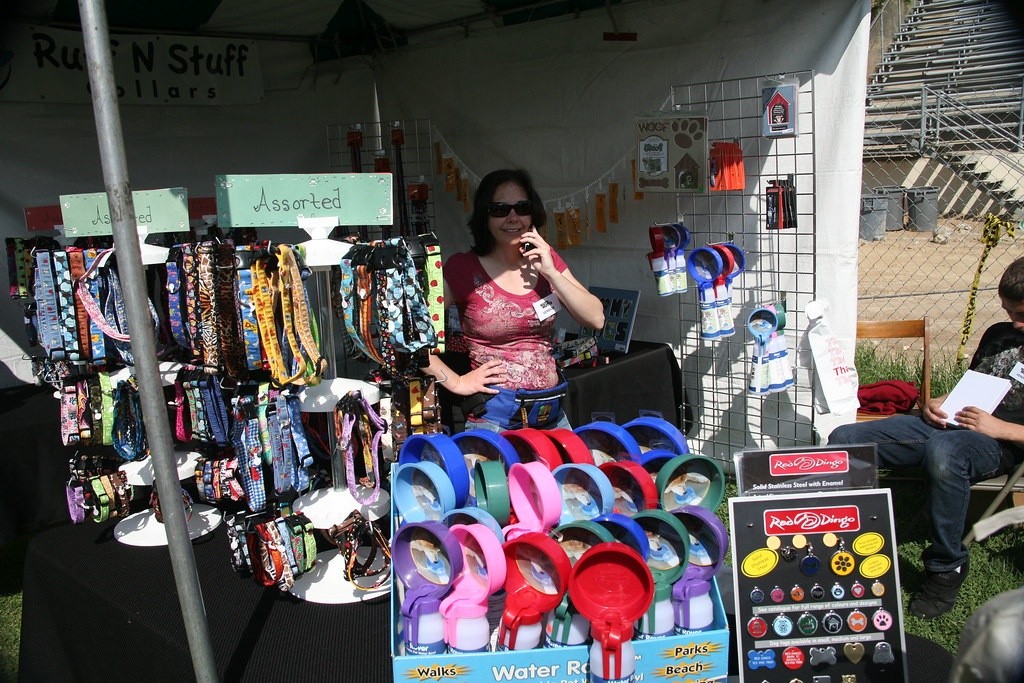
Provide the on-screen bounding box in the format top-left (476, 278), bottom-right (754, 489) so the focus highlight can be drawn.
top-left (910, 560), bottom-right (969, 619)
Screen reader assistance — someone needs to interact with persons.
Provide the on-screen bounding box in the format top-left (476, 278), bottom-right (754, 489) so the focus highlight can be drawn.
top-left (416, 170), bottom-right (605, 460)
top-left (827, 256), bottom-right (1024, 619)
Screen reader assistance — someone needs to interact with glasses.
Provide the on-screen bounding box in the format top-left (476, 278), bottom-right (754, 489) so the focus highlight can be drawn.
top-left (484, 201), bottom-right (533, 216)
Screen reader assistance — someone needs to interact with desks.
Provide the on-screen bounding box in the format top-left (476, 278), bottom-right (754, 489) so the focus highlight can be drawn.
top-left (439, 332), bottom-right (694, 438)
top-left (1, 383), bottom-right (396, 683)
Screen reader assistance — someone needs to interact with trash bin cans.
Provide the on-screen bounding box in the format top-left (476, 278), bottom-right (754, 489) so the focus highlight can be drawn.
top-left (873, 184), bottom-right (904, 232)
top-left (905, 186), bottom-right (941, 232)
top-left (859, 193), bottom-right (890, 242)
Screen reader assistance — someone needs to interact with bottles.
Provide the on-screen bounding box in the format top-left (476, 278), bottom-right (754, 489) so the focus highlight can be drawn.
top-left (749, 330), bottom-right (794, 396)
top-left (650, 249), bottom-right (687, 296)
top-left (698, 278), bottom-right (735, 340)
top-left (403, 574), bottom-right (712, 683)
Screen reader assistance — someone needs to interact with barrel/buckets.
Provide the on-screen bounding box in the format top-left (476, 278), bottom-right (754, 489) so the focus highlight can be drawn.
top-left (872, 186), bottom-right (907, 231)
top-left (906, 185), bottom-right (939, 232)
top-left (859, 194), bottom-right (888, 240)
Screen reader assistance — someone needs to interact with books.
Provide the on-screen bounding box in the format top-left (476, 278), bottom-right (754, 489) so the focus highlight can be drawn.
top-left (762, 84), bottom-right (799, 139)
top-left (937, 370), bottom-right (1013, 431)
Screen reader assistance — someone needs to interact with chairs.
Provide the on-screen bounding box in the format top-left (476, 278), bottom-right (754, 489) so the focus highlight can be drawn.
top-left (855, 316), bottom-right (930, 423)
top-left (905, 457), bottom-right (1024, 549)
